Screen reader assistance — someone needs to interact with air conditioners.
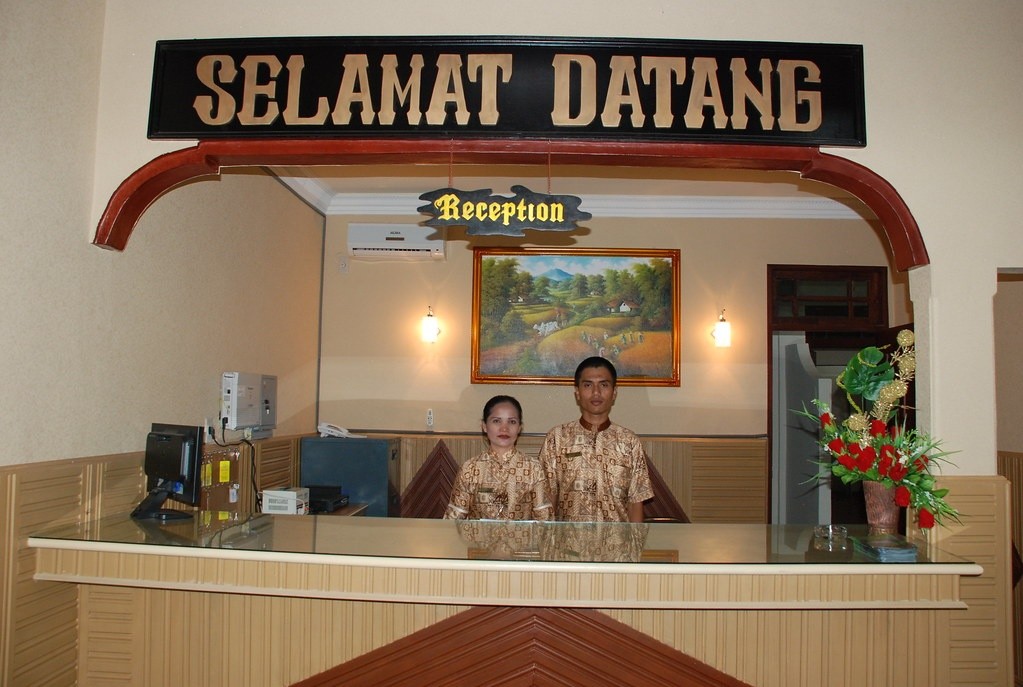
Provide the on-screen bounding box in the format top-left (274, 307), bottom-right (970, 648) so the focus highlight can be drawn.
top-left (347, 223), bottom-right (446, 262)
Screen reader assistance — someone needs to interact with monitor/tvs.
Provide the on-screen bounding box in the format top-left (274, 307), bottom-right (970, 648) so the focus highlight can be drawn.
top-left (130, 422), bottom-right (205, 520)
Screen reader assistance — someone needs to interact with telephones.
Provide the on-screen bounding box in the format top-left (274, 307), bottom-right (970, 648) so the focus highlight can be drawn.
top-left (318, 422), bottom-right (352, 438)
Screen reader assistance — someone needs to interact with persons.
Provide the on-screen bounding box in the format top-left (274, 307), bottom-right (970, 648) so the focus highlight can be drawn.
top-left (443, 395), bottom-right (556, 521)
top-left (456, 519), bottom-right (650, 562)
top-left (539, 356), bottom-right (654, 522)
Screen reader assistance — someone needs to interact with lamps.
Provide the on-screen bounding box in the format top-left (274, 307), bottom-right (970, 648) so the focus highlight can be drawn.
top-left (714, 308), bottom-right (732, 347)
top-left (421, 304), bottom-right (441, 343)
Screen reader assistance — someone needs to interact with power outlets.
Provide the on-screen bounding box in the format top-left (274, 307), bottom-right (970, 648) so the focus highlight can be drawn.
top-left (203, 419), bottom-right (215, 444)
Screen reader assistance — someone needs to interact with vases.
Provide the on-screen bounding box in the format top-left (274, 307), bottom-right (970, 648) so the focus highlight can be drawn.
top-left (863, 478), bottom-right (899, 528)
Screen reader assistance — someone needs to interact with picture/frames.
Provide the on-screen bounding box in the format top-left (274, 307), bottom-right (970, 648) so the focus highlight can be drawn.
top-left (470, 246), bottom-right (682, 387)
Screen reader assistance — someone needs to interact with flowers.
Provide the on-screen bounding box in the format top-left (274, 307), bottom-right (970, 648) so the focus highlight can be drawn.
top-left (796, 328), bottom-right (961, 531)
